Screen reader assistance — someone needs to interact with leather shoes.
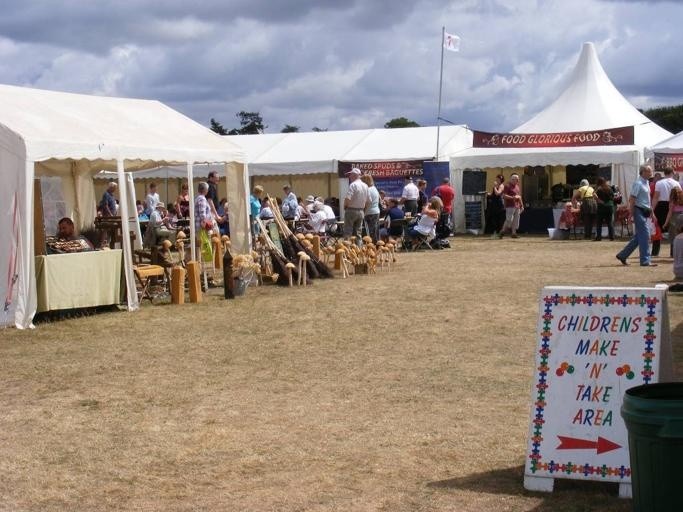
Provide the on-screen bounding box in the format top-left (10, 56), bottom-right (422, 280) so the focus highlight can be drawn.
top-left (616, 254), bottom-right (657, 266)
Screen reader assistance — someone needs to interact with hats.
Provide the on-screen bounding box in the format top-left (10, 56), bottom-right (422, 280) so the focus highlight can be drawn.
top-left (348, 168), bottom-right (361, 174)
top-left (306, 195), bottom-right (314, 202)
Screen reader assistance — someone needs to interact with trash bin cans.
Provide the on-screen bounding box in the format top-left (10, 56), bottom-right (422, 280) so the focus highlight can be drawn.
top-left (619, 381), bottom-right (683, 512)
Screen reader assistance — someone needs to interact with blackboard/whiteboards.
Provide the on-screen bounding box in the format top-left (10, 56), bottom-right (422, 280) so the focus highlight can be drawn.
top-left (465, 201), bottom-right (481, 229)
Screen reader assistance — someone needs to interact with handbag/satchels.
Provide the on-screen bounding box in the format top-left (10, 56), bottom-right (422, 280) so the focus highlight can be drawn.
top-left (640, 207), bottom-right (652, 217)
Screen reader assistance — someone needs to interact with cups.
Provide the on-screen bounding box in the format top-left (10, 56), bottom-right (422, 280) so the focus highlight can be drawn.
top-left (405, 212), bottom-right (411, 217)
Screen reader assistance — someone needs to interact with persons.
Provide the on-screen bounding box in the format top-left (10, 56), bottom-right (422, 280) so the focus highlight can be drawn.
top-left (572, 179), bottom-right (599, 240)
top-left (495, 174), bottom-right (524, 238)
top-left (94, 171), bottom-right (228, 252)
top-left (478, 175), bottom-right (505, 239)
top-left (54, 217), bottom-right (94, 251)
top-left (648, 168), bottom-right (683, 293)
top-left (594, 177), bottom-right (616, 241)
top-left (615, 164), bottom-right (659, 267)
top-left (249, 167), bottom-right (455, 249)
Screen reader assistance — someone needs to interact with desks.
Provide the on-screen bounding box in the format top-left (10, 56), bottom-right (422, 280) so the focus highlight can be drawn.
top-left (558, 207), bottom-right (632, 240)
top-left (34, 249), bottom-right (123, 314)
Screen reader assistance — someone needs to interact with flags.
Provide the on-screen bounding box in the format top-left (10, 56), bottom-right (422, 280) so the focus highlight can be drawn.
top-left (442, 32), bottom-right (459, 52)
